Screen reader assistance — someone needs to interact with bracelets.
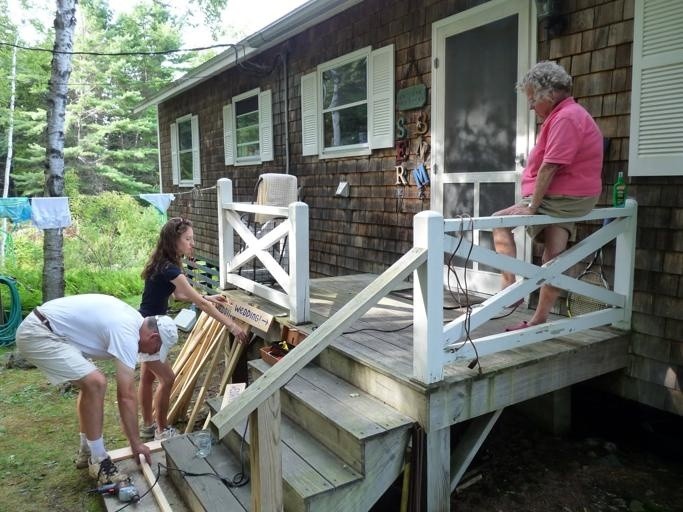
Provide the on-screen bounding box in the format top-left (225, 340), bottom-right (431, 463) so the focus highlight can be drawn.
top-left (203, 295), bottom-right (205, 299)
top-left (528, 203), bottom-right (537, 209)
top-left (230, 322), bottom-right (236, 335)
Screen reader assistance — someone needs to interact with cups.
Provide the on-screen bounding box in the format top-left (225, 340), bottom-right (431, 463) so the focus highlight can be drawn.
top-left (191, 426), bottom-right (212, 459)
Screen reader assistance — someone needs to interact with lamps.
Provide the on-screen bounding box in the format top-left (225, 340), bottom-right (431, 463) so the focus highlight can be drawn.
top-left (535, 0), bottom-right (559, 43)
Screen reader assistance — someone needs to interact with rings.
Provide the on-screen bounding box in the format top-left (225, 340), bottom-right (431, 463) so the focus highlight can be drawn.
top-left (221, 295), bottom-right (227, 298)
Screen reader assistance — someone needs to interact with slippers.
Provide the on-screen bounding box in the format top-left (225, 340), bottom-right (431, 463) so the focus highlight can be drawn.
top-left (506, 321), bottom-right (529, 331)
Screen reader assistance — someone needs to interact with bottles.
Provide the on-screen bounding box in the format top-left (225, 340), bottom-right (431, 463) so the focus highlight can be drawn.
top-left (612, 171), bottom-right (626, 209)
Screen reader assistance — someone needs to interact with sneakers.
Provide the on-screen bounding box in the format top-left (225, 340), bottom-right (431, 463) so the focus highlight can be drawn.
top-left (139, 423), bottom-right (180, 440)
top-left (76, 451), bottom-right (129, 483)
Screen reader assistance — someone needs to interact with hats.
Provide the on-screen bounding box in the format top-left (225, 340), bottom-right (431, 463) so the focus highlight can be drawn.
top-left (155, 315), bottom-right (178, 363)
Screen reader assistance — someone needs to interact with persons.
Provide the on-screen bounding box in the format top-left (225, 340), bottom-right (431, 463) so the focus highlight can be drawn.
top-left (138, 215), bottom-right (249, 442)
top-left (14, 292), bottom-right (178, 488)
top-left (487, 59), bottom-right (605, 333)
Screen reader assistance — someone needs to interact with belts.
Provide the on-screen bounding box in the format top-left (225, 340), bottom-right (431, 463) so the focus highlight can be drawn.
top-left (33, 307), bottom-right (52, 331)
